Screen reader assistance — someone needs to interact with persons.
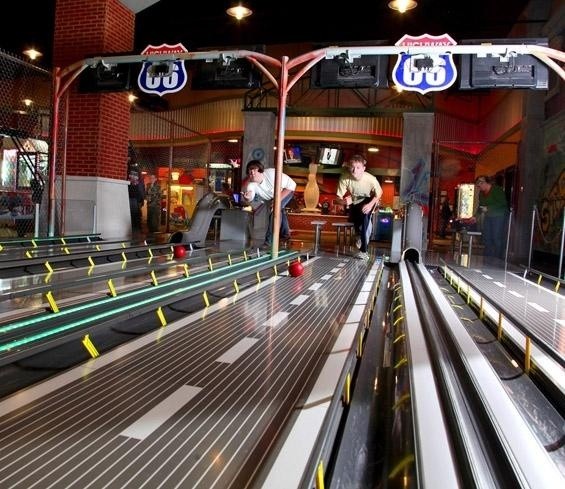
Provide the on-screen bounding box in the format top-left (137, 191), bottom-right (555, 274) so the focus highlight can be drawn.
top-left (438, 197), bottom-right (452, 239)
top-left (240, 160), bottom-right (298, 246)
top-left (128, 171), bottom-right (147, 232)
top-left (335, 153), bottom-right (383, 259)
top-left (474, 174), bottom-right (510, 256)
top-left (147, 174), bottom-right (161, 233)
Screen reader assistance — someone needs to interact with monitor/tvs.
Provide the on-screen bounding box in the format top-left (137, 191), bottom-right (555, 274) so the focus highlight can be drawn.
top-left (310, 40), bottom-right (390, 90)
top-left (459, 37), bottom-right (549, 91)
top-left (191, 44), bottom-right (266, 90)
top-left (283, 146), bottom-right (305, 165)
top-left (314, 146), bottom-right (343, 166)
top-left (78, 51), bottom-right (143, 93)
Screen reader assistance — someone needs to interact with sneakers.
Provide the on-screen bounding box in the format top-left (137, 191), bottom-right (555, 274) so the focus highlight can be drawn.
top-left (247, 247), bottom-right (263, 256)
top-left (354, 251), bottom-right (368, 259)
top-left (355, 239), bottom-right (362, 249)
